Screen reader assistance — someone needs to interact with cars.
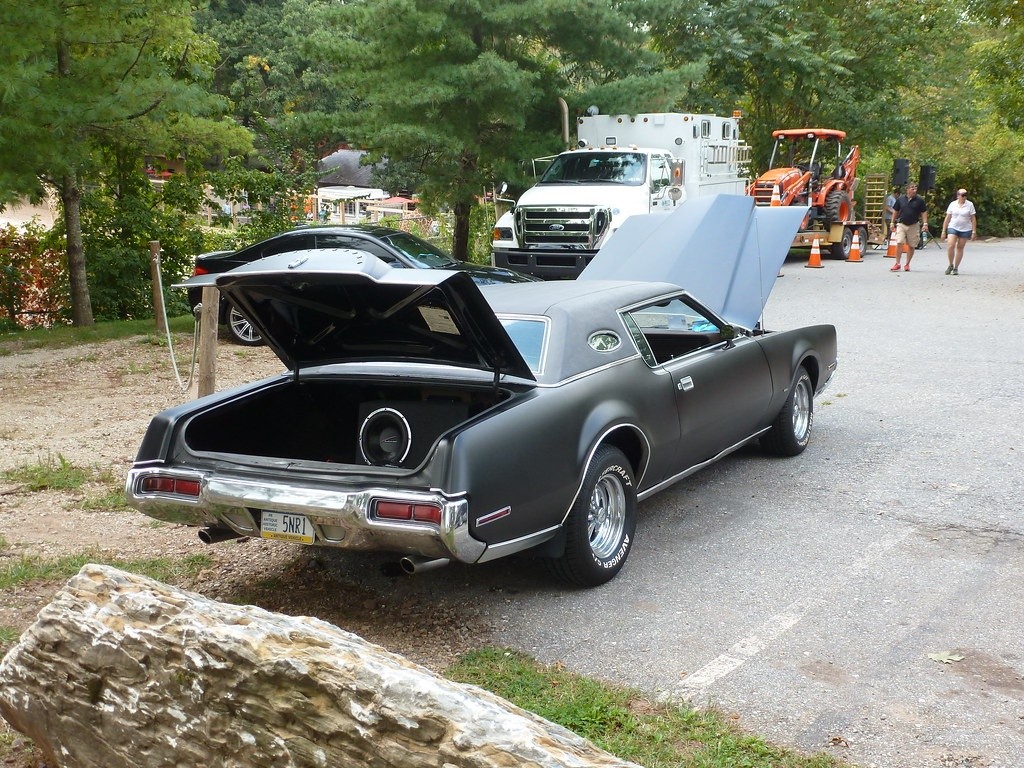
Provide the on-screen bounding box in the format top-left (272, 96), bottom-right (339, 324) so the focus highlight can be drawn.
top-left (119, 192), bottom-right (841, 593)
top-left (186, 223), bottom-right (549, 348)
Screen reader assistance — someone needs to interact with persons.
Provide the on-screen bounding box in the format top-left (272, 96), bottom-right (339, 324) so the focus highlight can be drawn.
top-left (942, 188), bottom-right (977, 275)
top-left (890, 182), bottom-right (929, 272)
top-left (883, 188), bottom-right (902, 249)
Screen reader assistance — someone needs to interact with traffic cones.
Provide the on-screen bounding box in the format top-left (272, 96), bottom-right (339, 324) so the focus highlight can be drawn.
top-left (884, 227), bottom-right (898, 258)
top-left (845, 229), bottom-right (864, 262)
top-left (902, 238), bottom-right (909, 253)
top-left (804, 233), bottom-right (824, 269)
top-left (770, 179), bottom-right (781, 207)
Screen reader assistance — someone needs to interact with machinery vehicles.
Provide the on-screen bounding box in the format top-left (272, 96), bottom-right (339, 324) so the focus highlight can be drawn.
top-left (745, 127), bottom-right (861, 233)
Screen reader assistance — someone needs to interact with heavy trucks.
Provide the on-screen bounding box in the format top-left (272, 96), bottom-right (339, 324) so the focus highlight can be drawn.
top-left (487, 106), bottom-right (890, 281)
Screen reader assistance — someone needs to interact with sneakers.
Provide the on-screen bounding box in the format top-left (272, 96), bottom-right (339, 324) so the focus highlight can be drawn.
top-left (904, 263), bottom-right (910, 271)
top-left (953, 268), bottom-right (959, 275)
top-left (891, 264), bottom-right (901, 270)
top-left (945, 265), bottom-right (953, 274)
